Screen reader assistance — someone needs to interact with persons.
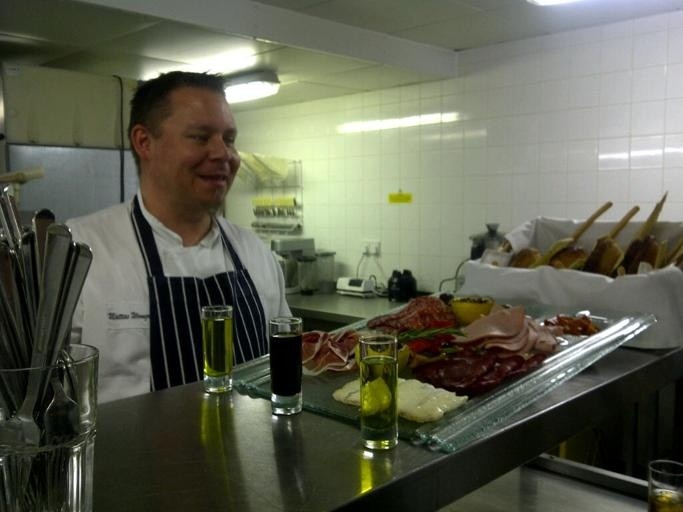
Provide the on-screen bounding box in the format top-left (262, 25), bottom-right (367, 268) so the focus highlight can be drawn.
top-left (53, 69), bottom-right (295, 408)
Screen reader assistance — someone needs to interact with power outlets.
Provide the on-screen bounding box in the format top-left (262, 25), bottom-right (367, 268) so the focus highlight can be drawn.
top-left (360, 241), bottom-right (380, 256)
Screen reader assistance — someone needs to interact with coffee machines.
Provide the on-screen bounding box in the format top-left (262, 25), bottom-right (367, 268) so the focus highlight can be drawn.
top-left (272, 237), bottom-right (319, 293)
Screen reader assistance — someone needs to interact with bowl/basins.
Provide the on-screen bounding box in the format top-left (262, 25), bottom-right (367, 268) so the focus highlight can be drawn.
top-left (452, 297), bottom-right (494, 322)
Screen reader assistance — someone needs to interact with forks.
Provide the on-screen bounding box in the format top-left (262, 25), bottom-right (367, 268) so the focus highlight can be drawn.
top-left (0, 193), bottom-right (93, 512)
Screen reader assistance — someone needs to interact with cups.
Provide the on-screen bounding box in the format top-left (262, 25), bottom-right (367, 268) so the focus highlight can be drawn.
top-left (648, 461), bottom-right (683, 512)
top-left (0, 344), bottom-right (98, 512)
top-left (357, 334), bottom-right (399, 449)
top-left (267, 316), bottom-right (305, 415)
top-left (200, 305), bottom-right (237, 393)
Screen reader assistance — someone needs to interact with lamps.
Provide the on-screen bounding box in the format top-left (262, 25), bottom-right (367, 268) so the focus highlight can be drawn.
top-left (225, 71), bottom-right (281, 104)
